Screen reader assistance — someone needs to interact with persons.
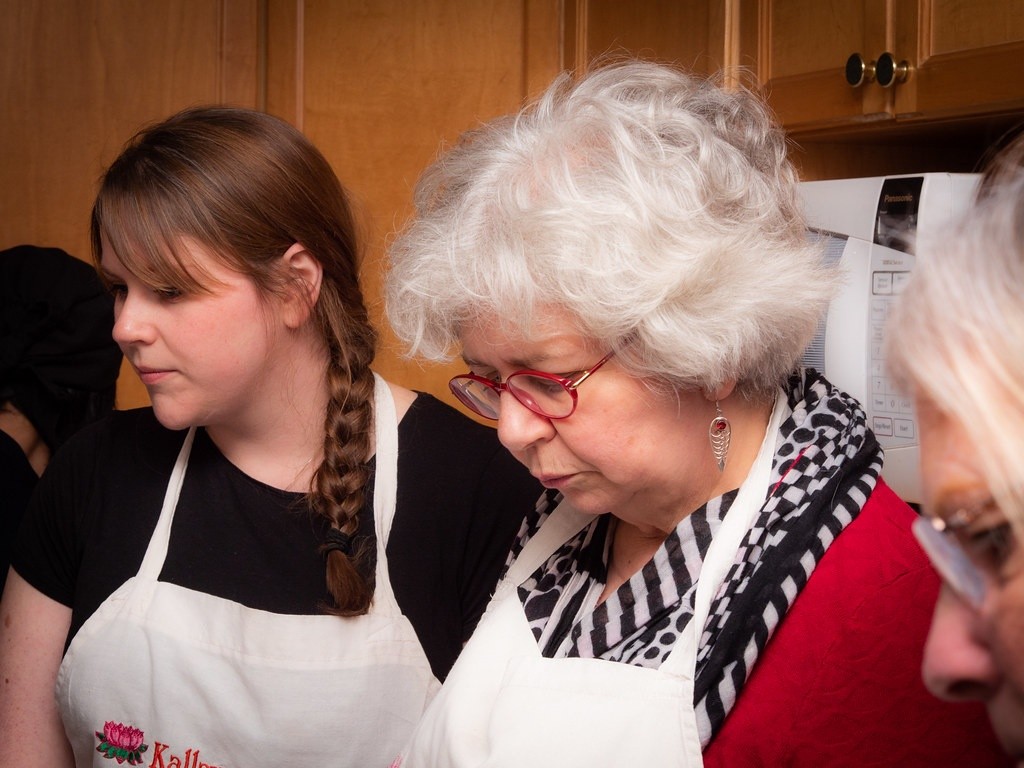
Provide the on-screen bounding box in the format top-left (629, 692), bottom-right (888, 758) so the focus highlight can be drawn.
top-left (0, 106), bottom-right (547, 768)
top-left (384, 61), bottom-right (1006, 768)
top-left (880, 138), bottom-right (1024, 768)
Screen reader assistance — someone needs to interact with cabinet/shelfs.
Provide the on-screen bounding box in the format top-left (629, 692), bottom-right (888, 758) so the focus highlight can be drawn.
top-left (563, 0), bottom-right (1024, 141)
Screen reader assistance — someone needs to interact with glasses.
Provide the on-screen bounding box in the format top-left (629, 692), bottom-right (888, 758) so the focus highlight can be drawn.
top-left (448, 333), bottom-right (640, 421)
top-left (909, 485), bottom-right (1024, 619)
